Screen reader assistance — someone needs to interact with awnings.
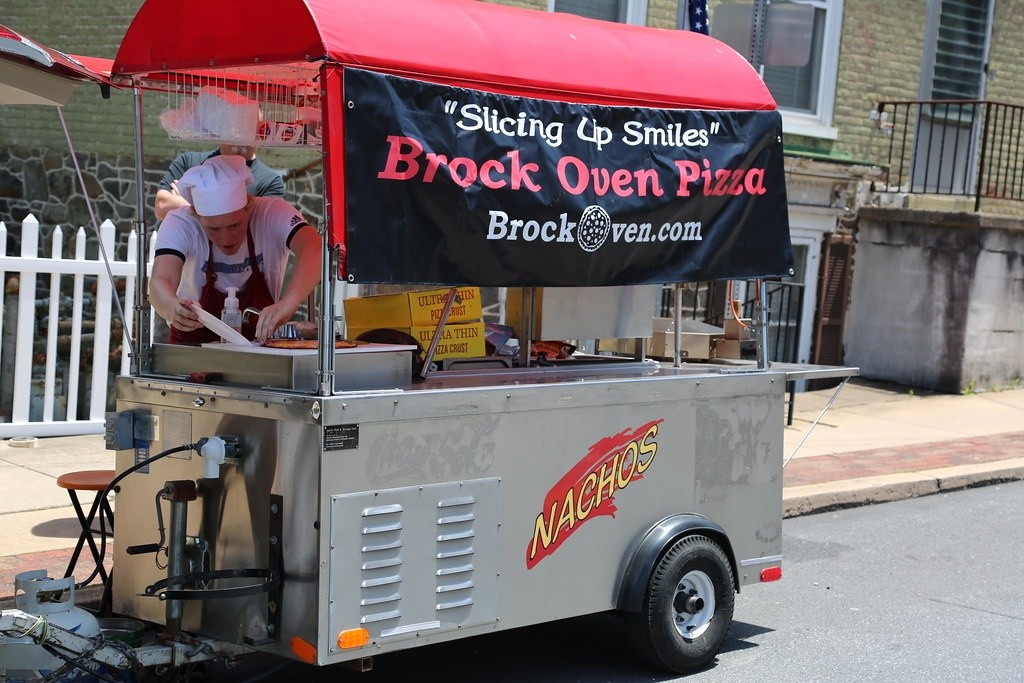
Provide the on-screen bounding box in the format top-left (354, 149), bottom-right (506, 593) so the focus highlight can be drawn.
top-left (0, 23), bottom-right (312, 346)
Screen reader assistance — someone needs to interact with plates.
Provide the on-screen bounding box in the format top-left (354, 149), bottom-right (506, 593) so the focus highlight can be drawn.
top-left (192, 303), bottom-right (254, 346)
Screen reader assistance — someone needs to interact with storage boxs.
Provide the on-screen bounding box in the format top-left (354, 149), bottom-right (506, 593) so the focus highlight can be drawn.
top-left (600, 331), bottom-right (710, 359)
top-left (343, 286), bottom-right (483, 328)
top-left (346, 320), bottom-right (486, 362)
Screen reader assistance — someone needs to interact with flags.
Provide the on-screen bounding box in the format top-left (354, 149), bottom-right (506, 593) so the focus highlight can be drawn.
top-left (684, 0), bottom-right (710, 37)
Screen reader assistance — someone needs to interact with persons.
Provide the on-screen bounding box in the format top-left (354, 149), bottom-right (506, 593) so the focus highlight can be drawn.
top-left (147, 154), bottom-right (323, 381)
top-left (154, 143), bottom-right (286, 220)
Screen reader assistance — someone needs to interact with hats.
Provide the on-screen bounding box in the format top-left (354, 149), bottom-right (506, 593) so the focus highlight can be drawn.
top-left (175, 155), bottom-right (255, 217)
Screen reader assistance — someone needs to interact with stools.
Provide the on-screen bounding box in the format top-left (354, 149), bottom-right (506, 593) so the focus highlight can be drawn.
top-left (55, 469), bottom-right (117, 602)
